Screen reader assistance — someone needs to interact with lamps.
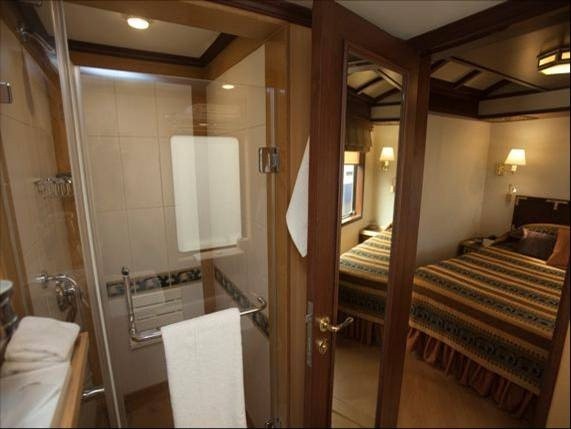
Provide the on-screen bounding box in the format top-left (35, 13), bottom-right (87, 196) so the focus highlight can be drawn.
top-left (535, 45), bottom-right (570, 76)
top-left (378, 146), bottom-right (395, 163)
top-left (503, 148), bottom-right (526, 173)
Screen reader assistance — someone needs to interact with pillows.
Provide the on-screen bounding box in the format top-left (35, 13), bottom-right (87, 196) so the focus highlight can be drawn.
top-left (516, 222), bottom-right (570, 235)
top-left (515, 226), bottom-right (555, 260)
top-left (546, 227), bottom-right (570, 269)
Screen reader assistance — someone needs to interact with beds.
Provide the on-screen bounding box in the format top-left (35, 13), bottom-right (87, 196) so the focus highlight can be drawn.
top-left (339, 217), bottom-right (394, 345)
top-left (411, 196), bottom-right (570, 418)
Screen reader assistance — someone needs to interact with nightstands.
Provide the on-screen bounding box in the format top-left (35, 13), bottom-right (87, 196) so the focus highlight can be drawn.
top-left (457, 235), bottom-right (502, 255)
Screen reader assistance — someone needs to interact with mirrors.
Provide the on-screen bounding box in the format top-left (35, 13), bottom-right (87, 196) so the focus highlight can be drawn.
top-left (326, 37), bottom-right (409, 426)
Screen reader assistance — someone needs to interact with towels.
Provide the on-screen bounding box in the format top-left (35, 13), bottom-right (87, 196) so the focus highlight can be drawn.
top-left (0, 312), bottom-right (82, 375)
top-left (160, 307), bottom-right (250, 429)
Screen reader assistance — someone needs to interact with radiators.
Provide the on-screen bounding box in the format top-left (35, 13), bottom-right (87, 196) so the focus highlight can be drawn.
top-left (128, 286), bottom-right (185, 349)
